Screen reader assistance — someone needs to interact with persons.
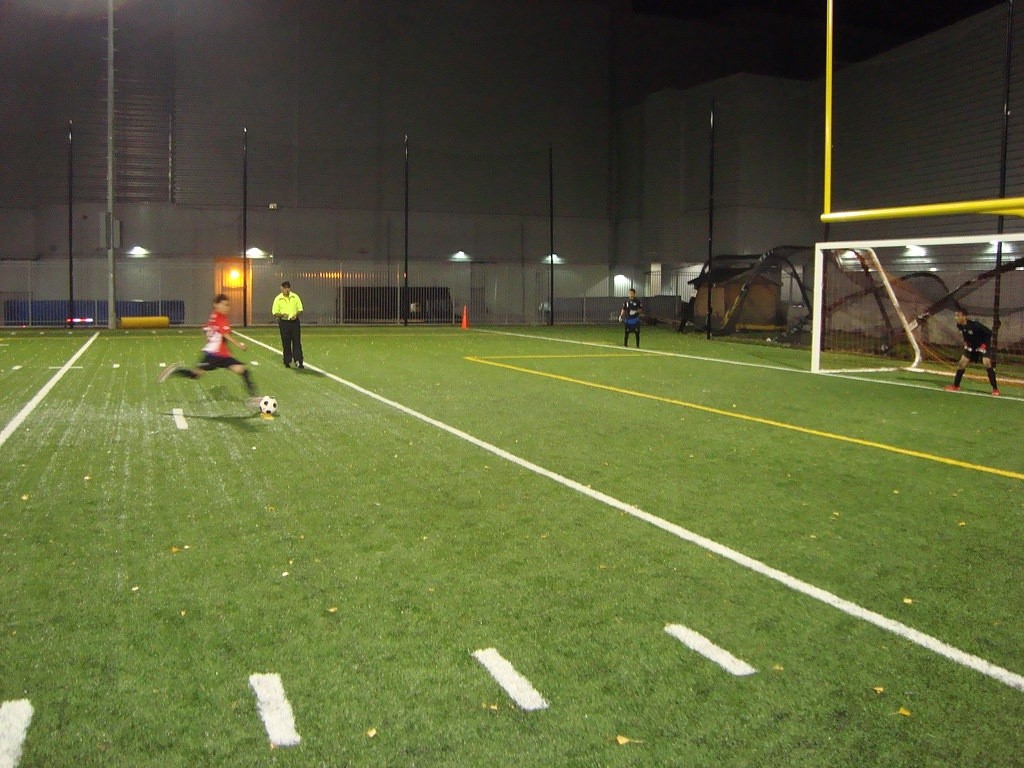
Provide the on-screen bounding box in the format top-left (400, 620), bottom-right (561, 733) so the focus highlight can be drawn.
top-left (272, 281), bottom-right (305, 368)
top-left (943, 309), bottom-right (1000, 396)
top-left (155, 293), bottom-right (263, 408)
top-left (619, 289), bottom-right (643, 348)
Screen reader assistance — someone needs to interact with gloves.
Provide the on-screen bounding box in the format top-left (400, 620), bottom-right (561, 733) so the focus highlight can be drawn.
top-left (975, 344), bottom-right (987, 354)
top-left (630, 310), bottom-right (637, 315)
top-left (963, 342), bottom-right (972, 351)
top-left (619, 316), bottom-right (623, 323)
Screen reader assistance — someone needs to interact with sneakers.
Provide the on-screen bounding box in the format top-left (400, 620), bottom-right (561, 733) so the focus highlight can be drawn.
top-left (944, 384), bottom-right (960, 392)
top-left (992, 388), bottom-right (1000, 397)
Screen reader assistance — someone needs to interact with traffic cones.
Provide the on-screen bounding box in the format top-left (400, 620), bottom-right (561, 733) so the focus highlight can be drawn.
top-left (459, 304), bottom-right (470, 329)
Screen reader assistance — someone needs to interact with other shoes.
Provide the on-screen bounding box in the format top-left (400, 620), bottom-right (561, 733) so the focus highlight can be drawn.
top-left (244, 397), bottom-right (264, 408)
top-left (158, 364), bottom-right (179, 382)
top-left (284, 362), bottom-right (290, 368)
top-left (294, 362), bottom-right (304, 370)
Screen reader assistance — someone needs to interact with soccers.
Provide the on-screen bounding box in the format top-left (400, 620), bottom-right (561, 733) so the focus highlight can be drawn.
top-left (259, 395), bottom-right (278, 415)
